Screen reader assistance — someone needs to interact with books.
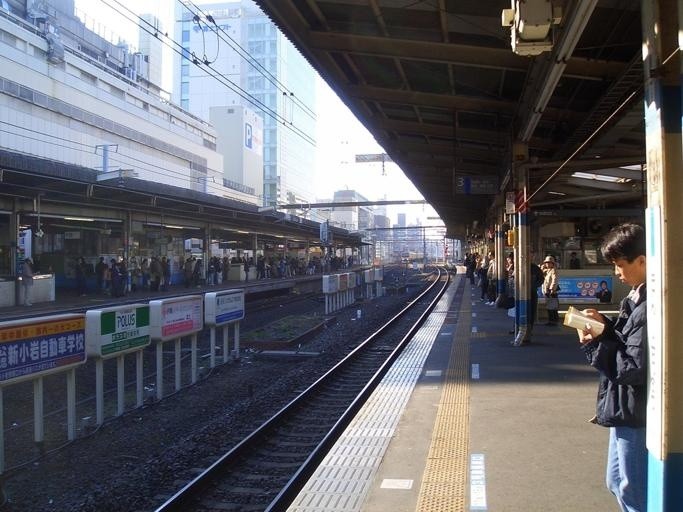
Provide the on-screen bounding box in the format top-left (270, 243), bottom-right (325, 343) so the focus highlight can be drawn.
top-left (563, 305), bottom-right (605, 336)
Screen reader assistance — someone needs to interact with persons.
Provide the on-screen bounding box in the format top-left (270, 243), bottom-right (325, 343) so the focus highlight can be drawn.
top-left (594, 280), bottom-right (611, 303)
top-left (574, 223), bottom-right (648, 512)
top-left (231, 251), bottom-right (353, 280)
top-left (569, 251), bottom-right (580, 269)
top-left (20, 255), bottom-right (37, 308)
top-left (48, 255), bottom-right (171, 296)
top-left (541, 256), bottom-right (560, 326)
top-left (183, 256), bottom-right (229, 286)
top-left (463, 250), bottom-right (513, 307)
top-left (530, 250), bottom-right (545, 338)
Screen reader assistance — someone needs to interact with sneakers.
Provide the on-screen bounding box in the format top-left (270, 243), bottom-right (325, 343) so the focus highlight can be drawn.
top-left (480, 299), bottom-right (496, 306)
top-left (545, 323), bottom-right (552, 326)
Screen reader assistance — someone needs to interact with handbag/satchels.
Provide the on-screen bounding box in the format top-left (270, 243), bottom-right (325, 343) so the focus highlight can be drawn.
top-left (478, 279), bottom-right (482, 288)
top-left (546, 297), bottom-right (558, 310)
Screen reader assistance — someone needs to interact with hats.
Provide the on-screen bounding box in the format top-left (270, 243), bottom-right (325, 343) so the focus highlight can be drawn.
top-left (543, 256), bottom-right (556, 264)
